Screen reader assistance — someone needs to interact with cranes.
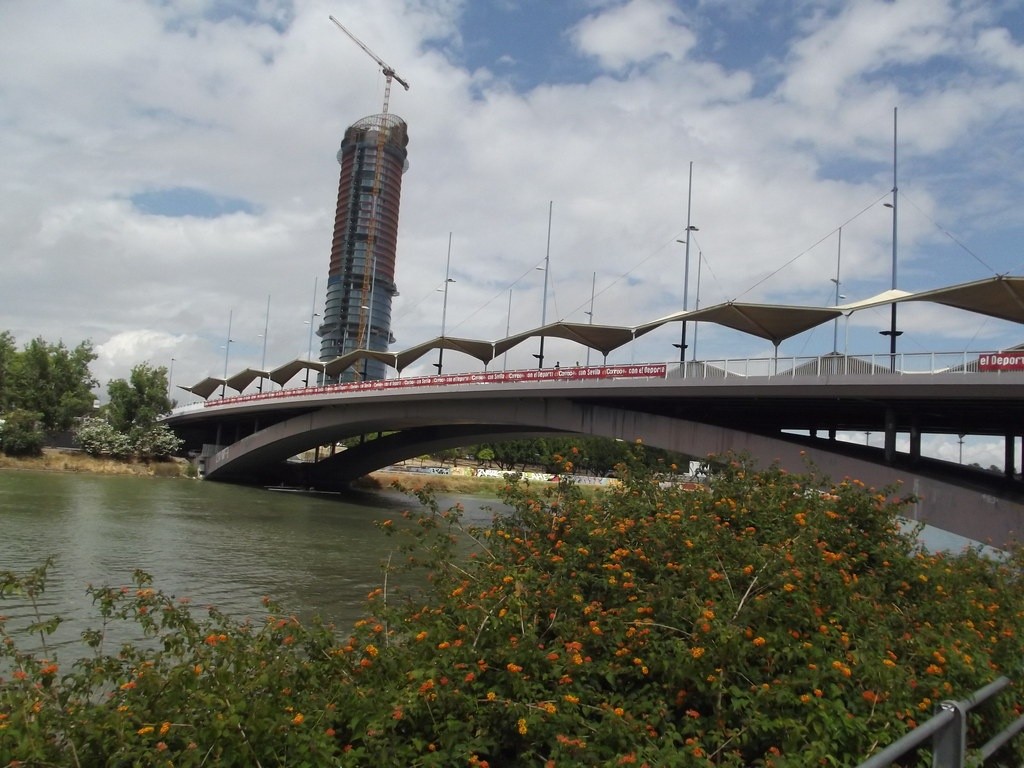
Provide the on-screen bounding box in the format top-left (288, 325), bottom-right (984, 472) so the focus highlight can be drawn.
top-left (329, 14), bottom-right (409, 383)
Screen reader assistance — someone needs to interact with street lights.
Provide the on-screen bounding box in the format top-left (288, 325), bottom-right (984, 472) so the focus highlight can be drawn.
top-left (168, 358), bottom-right (177, 402)
top-left (219, 310), bottom-right (235, 400)
top-left (302, 275), bottom-right (321, 388)
top-left (532, 200), bottom-right (553, 372)
top-left (584, 271), bottom-right (596, 366)
top-left (673, 160), bottom-right (699, 360)
top-left (257, 293), bottom-right (270, 394)
top-left (879, 107), bottom-right (904, 376)
top-left (831, 227), bottom-right (846, 352)
top-left (433, 231), bottom-right (456, 374)
